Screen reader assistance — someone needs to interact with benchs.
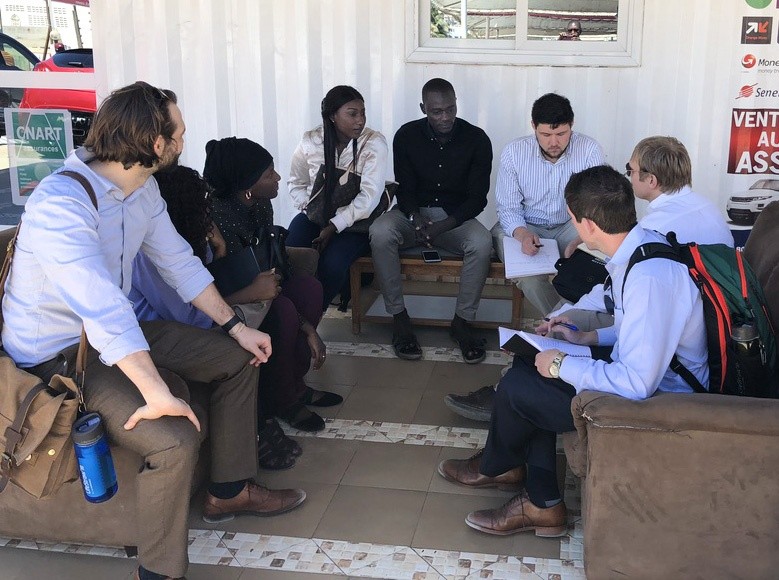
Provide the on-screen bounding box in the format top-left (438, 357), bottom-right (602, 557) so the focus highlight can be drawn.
top-left (350, 250), bottom-right (525, 340)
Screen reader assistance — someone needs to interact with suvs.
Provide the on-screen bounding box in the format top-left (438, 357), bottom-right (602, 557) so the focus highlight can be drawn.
top-left (0, 32), bottom-right (41, 137)
top-left (725, 179), bottom-right (779, 226)
top-left (17, 47), bottom-right (98, 159)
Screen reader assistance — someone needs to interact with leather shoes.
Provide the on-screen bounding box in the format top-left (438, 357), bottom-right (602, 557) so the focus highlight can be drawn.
top-left (465, 488), bottom-right (568, 538)
top-left (202, 479), bottom-right (306, 523)
top-left (263, 421), bottom-right (303, 456)
top-left (257, 435), bottom-right (296, 470)
top-left (437, 448), bottom-right (527, 492)
top-left (134, 564), bottom-right (186, 580)
top-left (391, 319), bottom-right (422, 359)
top-left (450, 321), bottom-right (486, 363)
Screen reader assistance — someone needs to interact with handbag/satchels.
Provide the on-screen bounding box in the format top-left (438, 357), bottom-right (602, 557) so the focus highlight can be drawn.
top-left (0, 351), bottom-right (86, 500)
top-left (307, 132), bottom-right (400, 234)
top-left (551, 249), bottom-right (612, 303)
top-left (233, 299), bottom-right (273, 329)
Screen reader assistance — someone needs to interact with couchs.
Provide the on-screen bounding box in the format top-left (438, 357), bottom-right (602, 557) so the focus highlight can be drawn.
top-left (565, 201), bottom-right (779, 580)
top-left (0, 227), bottom-right (320, 559)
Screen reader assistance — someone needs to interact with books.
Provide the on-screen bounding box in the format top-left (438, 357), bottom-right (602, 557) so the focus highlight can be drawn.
top-left (499, 327), bottom-right (592, 366)
top-left (502, 236), bottom-right (560, 280)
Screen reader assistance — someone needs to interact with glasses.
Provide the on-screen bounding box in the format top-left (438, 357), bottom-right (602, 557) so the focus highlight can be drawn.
top-left (626, 163), bottom-right (658, 179)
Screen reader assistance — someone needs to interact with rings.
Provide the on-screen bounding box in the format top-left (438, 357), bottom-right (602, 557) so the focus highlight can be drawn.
top-left (322, 356), bottom-right (326, 357)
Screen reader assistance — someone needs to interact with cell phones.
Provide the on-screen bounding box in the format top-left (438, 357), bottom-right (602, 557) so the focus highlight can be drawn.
top-left (421, 250), bottom-right (441, 263)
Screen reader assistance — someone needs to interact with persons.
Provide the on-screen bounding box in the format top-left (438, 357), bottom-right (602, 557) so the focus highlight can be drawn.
top-left (284, 85), bottom-right (389, 315)
top-left (437, 165), bottom-right (713, 537)
top-left (0, 80), bottom-right (306, 580)
top-left (443, 135), bottom-right (734, 423)
top-left (370, 78), bottom-right (493, 364)
top-left (128, 166), bottom-right (303, 471)
top-left (204, 135), bottom-right (342, 430)
top-left (493, 93), bottom-right (606, 319)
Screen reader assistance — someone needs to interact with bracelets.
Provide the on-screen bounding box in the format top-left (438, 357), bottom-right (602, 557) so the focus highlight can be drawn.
top-left (231, 324), bottom-right (245, 337)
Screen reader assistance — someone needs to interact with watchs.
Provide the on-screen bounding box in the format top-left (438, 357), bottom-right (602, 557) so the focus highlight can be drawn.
top-left (221, 313), bottom-right (244, 332)
top-left (548, 352), bottom-right (567, 379)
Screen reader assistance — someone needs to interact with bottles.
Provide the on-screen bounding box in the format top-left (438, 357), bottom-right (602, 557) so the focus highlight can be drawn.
top-left (731, 320), bottom-right (763, 357)
top-left (73, 411), bottom-right (118, 503)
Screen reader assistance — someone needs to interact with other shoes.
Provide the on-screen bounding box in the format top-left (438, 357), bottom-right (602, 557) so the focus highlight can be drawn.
top-left (298, 384), bottom-right (343, 406)
top-left (272, 400), bottom-right (325, 431)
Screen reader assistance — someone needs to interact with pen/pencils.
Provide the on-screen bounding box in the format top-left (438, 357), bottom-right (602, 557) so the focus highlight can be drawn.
top-left (543, 318), bottom-right (579, 331)
top-left (533, 244), bottom-right (543, 247)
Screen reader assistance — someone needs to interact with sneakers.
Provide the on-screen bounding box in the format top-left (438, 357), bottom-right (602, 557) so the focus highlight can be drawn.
top-left (444, 384), bottom-right (498, 421)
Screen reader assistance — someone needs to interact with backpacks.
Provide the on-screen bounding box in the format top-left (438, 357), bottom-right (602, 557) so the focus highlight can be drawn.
top-left (621, 231), bottom-right (779, 398)
